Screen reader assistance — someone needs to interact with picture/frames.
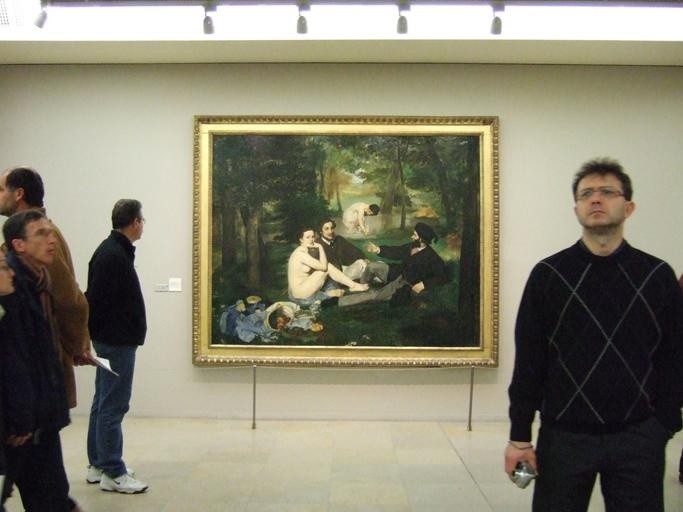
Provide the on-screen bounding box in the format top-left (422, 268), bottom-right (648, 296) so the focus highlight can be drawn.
top-left (192, 114), bottom-right (500, 370)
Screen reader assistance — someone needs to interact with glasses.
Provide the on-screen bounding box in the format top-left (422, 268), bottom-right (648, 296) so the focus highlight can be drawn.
top-left (573, 186), bottom-right (626, 201)
top-left (138, 218), bottom-right (146, 223)
top-left (0, 258), bottom-right (11, 271)
top-left (21, 229), bottom-right (55, 239)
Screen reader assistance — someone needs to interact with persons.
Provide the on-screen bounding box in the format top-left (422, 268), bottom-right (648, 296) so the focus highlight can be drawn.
top-left (85, 200), bottom-right (148, 494)
top-left (501, 159), bottom-right (682, 511)
top-left (0, 167), bottom-right (97, 432)
top-left (1, 246), bottom-right (16, 306)
top-left (237, 201), bottom-right (448, 336)
top-left (1, 207), bottom-right (78, 512)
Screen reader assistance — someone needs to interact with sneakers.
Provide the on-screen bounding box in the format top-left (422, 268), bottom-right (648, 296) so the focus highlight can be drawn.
top-left (320, 296), bottom-right (337, 307)
top-left (85, 464), bottom-right (135, 483)
top-left (100, 470), bottom-right (147, 494)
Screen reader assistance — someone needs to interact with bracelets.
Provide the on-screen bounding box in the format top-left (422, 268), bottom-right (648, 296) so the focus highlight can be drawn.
top-left (509, 441), bottom-right (533, 451)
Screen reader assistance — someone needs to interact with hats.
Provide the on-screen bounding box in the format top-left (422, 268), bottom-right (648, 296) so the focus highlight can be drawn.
top-left (415, 222), bottom-right (437, 244)
